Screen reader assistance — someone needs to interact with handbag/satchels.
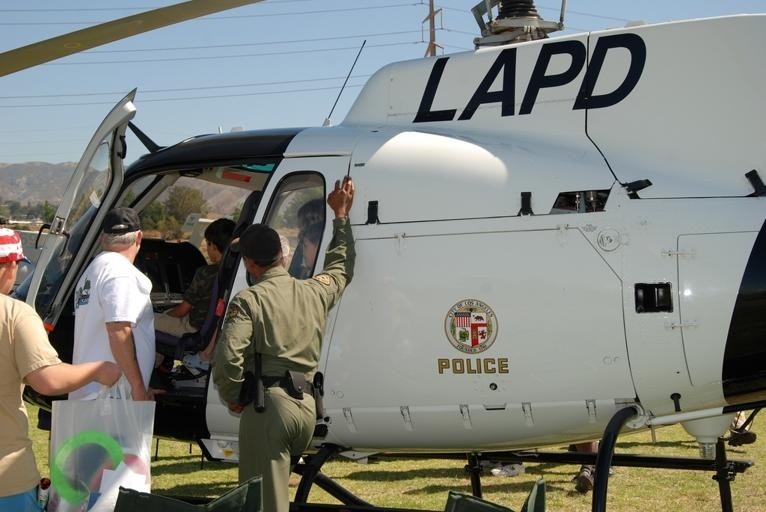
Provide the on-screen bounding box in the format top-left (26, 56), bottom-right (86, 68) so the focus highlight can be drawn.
top-left (48, 373), bottom-right (157, 512)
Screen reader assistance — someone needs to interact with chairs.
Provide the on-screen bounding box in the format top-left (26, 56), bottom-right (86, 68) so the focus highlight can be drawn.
top-left (155, 190), bottom-right (259, 361)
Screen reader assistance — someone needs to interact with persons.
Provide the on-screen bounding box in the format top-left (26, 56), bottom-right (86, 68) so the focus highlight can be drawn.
top-left (0, 226), bottom-right (121, 512)
top-left (67, 205), bottom-right (166, 402)
top-left (152, 218), bottom-right (239, 370)
top-left (297, 225), bottom-right (322, 268)
top-left (294, 195), bottom-right (321, 232)
top-left (729, 411), bottom-right (756, 446)
top-left (462, 451), bottom-right (527, 476)
top-left (212, 176), bottom-right (357, 512)
top-left (573, 439), bottom-right (615, 493)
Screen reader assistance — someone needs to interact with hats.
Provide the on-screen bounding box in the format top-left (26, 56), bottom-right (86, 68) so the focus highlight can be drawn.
top-left (0, 228), bottom-right (32, 265)
top-left (229, 223), bottom-right (282, 259)
top-left (103, 207), bottom-right (141, 233)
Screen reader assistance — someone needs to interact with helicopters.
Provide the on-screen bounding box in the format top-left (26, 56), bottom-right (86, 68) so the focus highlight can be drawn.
top-left (0, 0), bottom-right (766, 511)
